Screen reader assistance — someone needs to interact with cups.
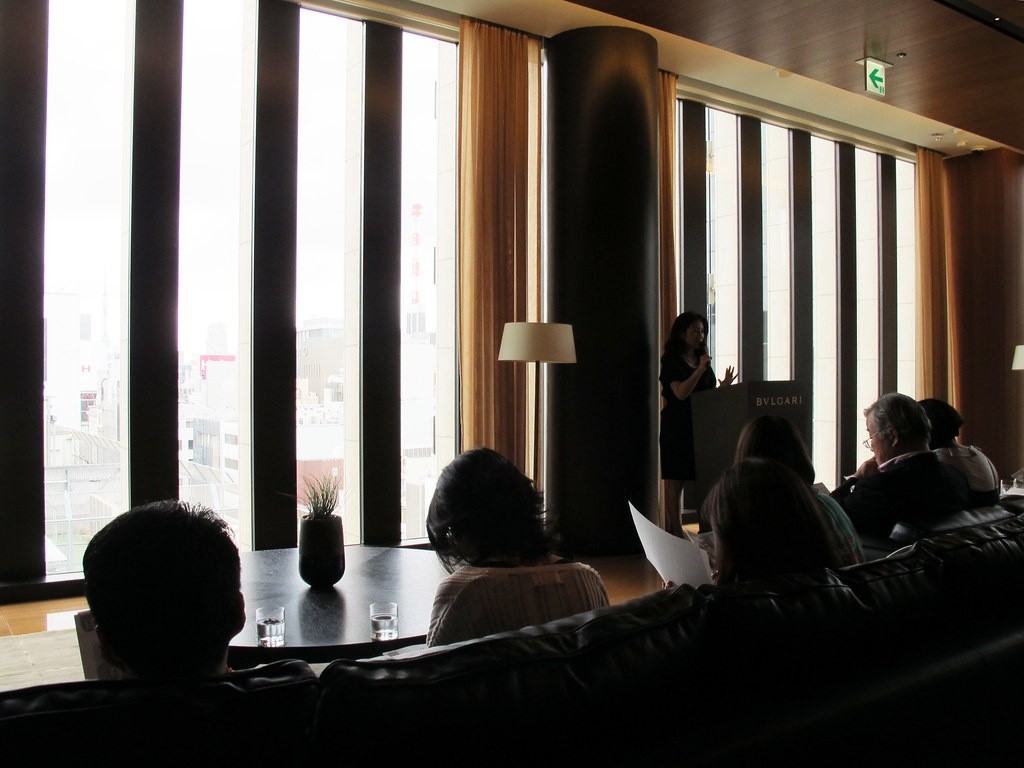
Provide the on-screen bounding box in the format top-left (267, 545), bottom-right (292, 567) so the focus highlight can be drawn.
top-left (256, 607), bottom-right (285, 647)
top-left (371, 603), bottom-right (399, 641)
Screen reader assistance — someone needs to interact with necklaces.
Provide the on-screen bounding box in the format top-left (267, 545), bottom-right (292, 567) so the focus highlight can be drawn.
top-left (681, 354), bottom-right (696, 366)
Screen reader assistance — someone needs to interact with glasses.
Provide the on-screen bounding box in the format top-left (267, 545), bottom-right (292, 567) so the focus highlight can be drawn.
top-left (863, 431), bottom-right (881, 448)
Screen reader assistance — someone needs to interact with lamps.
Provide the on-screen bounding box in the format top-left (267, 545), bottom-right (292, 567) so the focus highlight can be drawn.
top-left (497, 321), bottom-right (577, 490)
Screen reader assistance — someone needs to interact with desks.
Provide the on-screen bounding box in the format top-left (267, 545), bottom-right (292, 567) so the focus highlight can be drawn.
top-left (227, 547), bottom-right (461, 664)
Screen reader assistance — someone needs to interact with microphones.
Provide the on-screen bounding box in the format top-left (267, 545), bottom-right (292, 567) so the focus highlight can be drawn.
top-left (699, 342), bottom-right (710, 368)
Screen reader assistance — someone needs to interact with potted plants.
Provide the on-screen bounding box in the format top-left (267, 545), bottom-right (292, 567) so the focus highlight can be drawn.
top-left (299, 478), bottom-right (345, 591)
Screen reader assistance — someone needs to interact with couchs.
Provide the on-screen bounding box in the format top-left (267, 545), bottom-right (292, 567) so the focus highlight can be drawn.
top-left (0, 494), bottom-right (1024, 767)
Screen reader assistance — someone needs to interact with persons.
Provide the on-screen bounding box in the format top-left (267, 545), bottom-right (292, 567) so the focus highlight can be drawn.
top-left (828, 396), bottom-right (998, 523)
top-left (84, 499), bottom-right (244, 676)
top-left (428, 448), bottom-right (608, 646)
top-left (664, 413), bottom-right (864, 587)
top-left (659, 311), bottom-right (739, 535)
